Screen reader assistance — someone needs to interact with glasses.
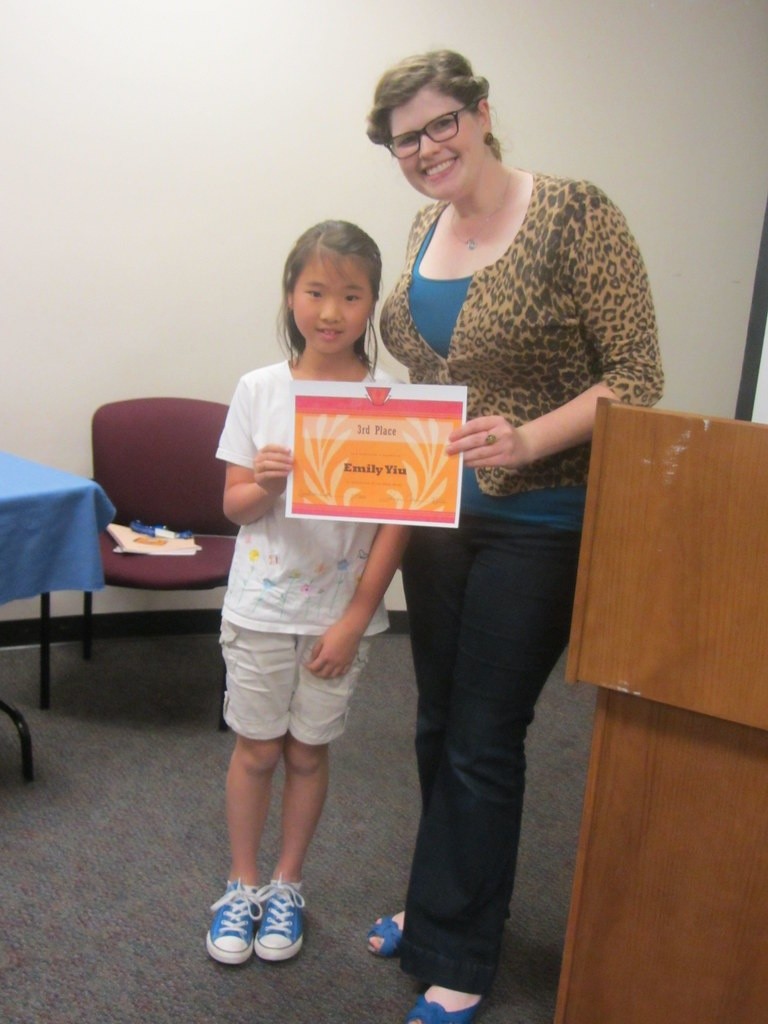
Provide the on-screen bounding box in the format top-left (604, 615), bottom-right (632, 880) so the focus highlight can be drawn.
top-left (384, 106), bottom-right (469, 159)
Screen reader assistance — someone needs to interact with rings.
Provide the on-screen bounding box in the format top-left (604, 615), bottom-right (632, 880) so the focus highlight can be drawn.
top-left (484, 430), bottom-right (496, 445)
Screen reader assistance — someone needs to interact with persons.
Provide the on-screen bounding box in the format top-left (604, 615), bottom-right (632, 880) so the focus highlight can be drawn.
top-left (365, 47), bottom-right (666, 1024)
top-left (205, 220), bottom-right (413, 966)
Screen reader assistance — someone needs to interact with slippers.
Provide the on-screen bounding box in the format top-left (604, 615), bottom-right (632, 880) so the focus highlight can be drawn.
top-left (404, 984), bottom-right (484, 1024)
top-left (366, 915), bottom-right (403, 959)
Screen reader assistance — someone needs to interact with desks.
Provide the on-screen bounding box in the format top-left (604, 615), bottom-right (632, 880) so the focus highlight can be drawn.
top-left (0, 450), bottom-right (118, 785)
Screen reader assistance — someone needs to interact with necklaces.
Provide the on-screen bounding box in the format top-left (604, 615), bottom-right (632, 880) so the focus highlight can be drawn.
top-left (451, 168), bottom-right (514, 250)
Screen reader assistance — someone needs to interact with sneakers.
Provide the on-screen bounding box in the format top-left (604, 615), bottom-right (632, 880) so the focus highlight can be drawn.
top-left (254, 883), bottom-right (302, 961)
top-left (206, 880), bottom-right (259, 964)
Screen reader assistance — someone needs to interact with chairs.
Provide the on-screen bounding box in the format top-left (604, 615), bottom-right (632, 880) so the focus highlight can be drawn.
top-left (39, 398), bottom-right (242, 732)
